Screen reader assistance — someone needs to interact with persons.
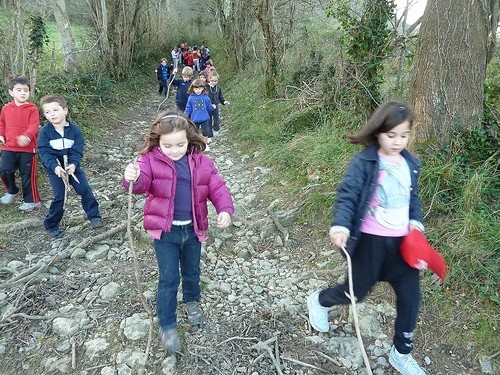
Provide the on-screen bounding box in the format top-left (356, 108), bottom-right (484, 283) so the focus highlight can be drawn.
top-left (183, 79), bottom-right (217, 151)
top-left (204, 71), bottom-right (230, 137)
top-left (170, 66), bottom-right (195, 120)
top-left (37, 93), bottom-right (104, 239)
top-left (122, 109), bottom-right (234, 352)
top-left (154, 57), bottom-right (174, 98)
top-left (170, 42), bottom-right (213, 86)
top-left (307, 102), bottom-right (426, 375)
top-left (0, 75), bottom-right (42, 211)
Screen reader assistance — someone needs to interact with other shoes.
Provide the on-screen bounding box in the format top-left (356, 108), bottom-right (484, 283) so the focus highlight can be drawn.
top-left (48, 226), bottom-right (65, 239)
top-left (89, 217), bottom-right (104, 228)
top-left (0, 191), bottom-right (20, 204)
top-left (212, 128), bottom-right (219, 136)
top-left (207, 138), bottom-right (212, 143)
top-left (205, 144), bottom-right (210, 151)
top-left (18, 201), bottom-right (42, 212)
top-left (185, 301), bottom-right (202, 324)
top-left (158, 325), bottom-right (181, 352)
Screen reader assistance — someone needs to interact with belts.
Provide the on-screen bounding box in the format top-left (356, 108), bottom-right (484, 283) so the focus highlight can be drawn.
top-left (171, 219), bottom-right (193, 226)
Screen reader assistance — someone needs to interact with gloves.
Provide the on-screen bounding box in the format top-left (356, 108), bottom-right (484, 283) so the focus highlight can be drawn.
top-left (223, 101), bottom-right (230, 106)
top-left (212, 104), bottom-right (216, 109)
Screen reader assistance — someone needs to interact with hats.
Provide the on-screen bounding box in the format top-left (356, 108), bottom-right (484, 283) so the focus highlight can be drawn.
top-left (400, 229), bottom-right (446, 279)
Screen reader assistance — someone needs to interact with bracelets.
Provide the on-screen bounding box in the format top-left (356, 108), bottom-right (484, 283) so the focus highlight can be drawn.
top-left (25, 135), bottom-right (31, 142)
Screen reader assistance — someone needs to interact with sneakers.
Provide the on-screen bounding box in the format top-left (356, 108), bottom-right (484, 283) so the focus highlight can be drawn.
top-left (389, 345), bottom-right (427, 375)
top-left (307, 291), bottom-right (330, 333)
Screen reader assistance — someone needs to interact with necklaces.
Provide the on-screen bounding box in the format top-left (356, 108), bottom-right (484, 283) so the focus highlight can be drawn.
top-left (210, 84), bottom-right (218, 94)
